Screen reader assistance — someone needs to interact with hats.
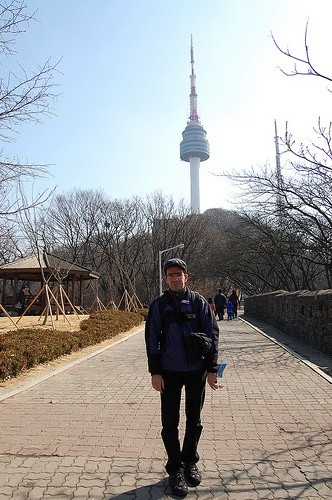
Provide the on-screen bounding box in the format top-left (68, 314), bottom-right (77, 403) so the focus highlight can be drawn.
top-left (164, 257), bottom-right (187, 273)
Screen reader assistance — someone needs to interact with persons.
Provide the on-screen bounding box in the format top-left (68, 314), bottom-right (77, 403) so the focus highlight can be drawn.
top-left (145, 258), bottom-right (220, 499)
top-left (224, 300), bottom-right (233, 320)
top-left (207, 297), bottom-right (215, 314)
top-left (214, 289), bottom-right (227, 321)
top-left (229, 289), bottom-right (240, 319)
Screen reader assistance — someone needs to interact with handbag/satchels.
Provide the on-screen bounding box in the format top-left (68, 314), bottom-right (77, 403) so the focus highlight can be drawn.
top-left (186, 332), bottom-right (212, 358)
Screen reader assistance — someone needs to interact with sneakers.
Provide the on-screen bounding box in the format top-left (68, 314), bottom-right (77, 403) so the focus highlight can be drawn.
top-left (182, 461), bottom-right (201, 486)
top-left (168, 468), bottom-right (188, 497)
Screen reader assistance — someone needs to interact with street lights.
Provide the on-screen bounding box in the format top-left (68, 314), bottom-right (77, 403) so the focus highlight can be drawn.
top-left (159, 243), bottom-right (186, 298)
